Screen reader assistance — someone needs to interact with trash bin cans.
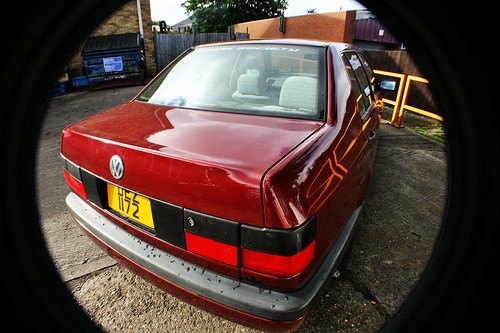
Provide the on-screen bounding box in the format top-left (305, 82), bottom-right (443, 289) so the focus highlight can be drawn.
top-left (55, 81), bottom-right (65, 96)
top-left (72, 75), bottom-right (88, 91)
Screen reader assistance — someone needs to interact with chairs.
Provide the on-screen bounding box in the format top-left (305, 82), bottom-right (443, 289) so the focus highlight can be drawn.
top-left (232, 74), bottom-right (280, 105)
top-left (277, 75), bottom-right (321, 114)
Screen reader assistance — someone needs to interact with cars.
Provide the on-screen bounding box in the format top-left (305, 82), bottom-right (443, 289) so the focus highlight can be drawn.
top-left (59, 37), bottom-right (398, 332)
